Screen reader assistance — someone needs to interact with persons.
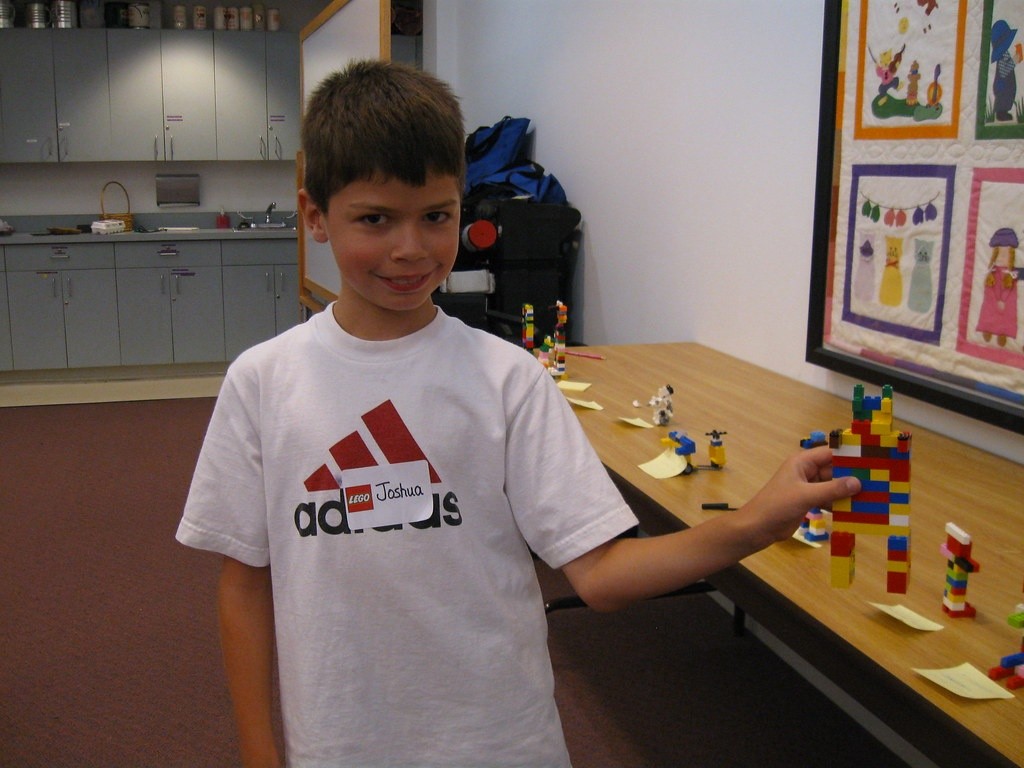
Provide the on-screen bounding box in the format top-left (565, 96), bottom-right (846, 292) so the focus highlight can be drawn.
top-left (176, 58), bottom-right (862, 767)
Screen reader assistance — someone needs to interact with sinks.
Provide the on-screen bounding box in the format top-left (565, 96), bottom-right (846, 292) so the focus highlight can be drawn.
top-left (231, 227), bottom-right (296, 232)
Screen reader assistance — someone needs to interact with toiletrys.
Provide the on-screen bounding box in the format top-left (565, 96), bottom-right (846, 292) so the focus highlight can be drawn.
top-left (216, 209), bottom-right (230, 228)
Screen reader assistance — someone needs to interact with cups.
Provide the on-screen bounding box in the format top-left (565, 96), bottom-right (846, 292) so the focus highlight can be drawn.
top-left (105, 2), bottom-right (129, 28)
top-left (128, 2), bottom-right (151, 29)
top-left (0, 0), bottom-right (15, 28)
top-left (79, 0), bottom-right (104, 27)
top-left (26, 3), bottom-right (52, 28)
top-left (51, 0), bottom-right (77, 28)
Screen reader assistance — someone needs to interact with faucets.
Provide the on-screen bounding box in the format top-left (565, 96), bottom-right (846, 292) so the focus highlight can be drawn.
top-left (264, 202), bottom-right (276, 223)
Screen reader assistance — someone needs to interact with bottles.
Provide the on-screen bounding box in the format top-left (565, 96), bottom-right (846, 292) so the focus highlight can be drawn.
top-left (172, 4), bottom-right (280, 31)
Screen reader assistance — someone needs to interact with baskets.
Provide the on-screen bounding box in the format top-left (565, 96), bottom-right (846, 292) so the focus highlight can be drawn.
top-left (101, 181), bottom-right (132, 231)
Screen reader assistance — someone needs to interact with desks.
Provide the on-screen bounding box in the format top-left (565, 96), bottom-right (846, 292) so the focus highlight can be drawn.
top-left (533, 343), bottom-right (1024, 768)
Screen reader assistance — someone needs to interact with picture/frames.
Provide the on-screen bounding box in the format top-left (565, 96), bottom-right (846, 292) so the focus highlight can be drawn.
top-left (805, 0), bottom-right (1024, 433)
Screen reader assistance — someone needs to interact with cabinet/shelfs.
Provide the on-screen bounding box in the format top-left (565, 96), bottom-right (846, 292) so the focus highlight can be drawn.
top-left (0, 238), bottom-right (306, 370)
top-left (1, 28), bottom-right (306, 163)
